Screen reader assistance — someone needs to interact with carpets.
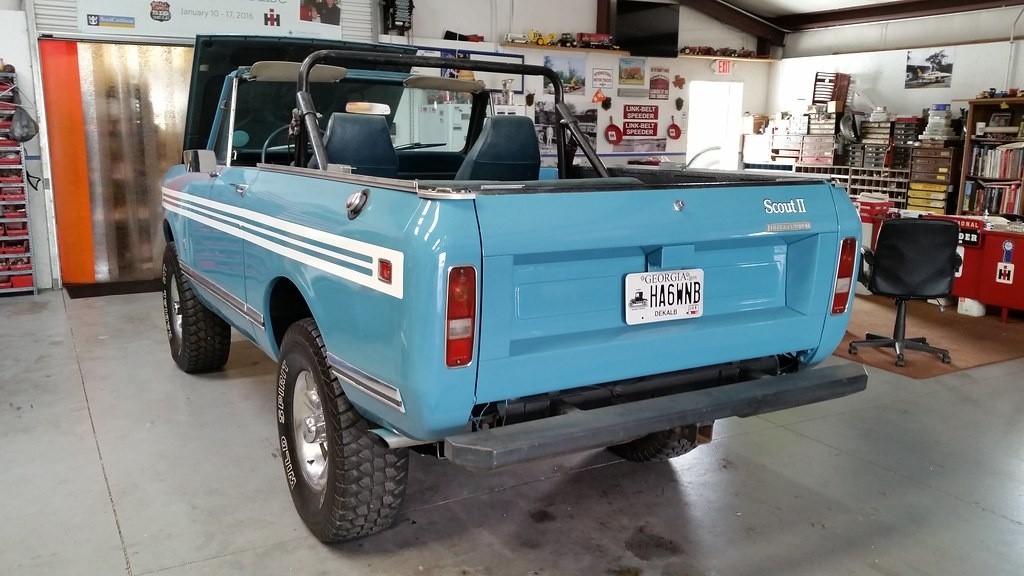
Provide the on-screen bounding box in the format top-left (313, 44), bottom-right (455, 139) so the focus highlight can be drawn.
top-left (832, 292), bottom-right (1024, 379)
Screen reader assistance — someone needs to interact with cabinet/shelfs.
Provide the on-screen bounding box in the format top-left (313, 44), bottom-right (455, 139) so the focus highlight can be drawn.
top-left (739, 71), bottom-right (1024, 323)
top-left (0, 71), bottom-right (39, 296)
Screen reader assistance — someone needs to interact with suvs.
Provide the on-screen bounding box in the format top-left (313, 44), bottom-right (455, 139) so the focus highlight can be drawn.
top-left (159, 33), bottom-right (868, 552)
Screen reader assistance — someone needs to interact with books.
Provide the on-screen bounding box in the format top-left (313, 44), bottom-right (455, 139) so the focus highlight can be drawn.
top-left (971, 144), bottom-right (1024, 179)
top-left (962, 180), bottom-right (1024, 216)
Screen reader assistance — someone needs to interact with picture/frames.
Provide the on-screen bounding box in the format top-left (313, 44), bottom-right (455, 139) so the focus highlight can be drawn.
top-left (989, 112), bottom-right (1010, 127)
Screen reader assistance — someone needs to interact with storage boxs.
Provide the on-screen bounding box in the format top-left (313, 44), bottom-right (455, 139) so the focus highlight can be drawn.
top-left (958, 295), bottom-right (987, 318)
top-left (0, 84), bottom-right (34, 290)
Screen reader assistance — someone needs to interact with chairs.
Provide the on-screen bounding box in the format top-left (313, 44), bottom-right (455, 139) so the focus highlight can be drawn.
top-left (305, 113), bottom-right (400, 174)
top-left (849, 217), bottom-right (963, 368)
top-left (454, 116), bottom-right (541, 181)
